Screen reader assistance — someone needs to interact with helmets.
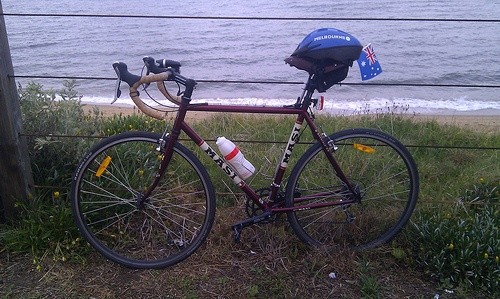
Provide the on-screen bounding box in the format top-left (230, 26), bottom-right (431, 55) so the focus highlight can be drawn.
top-left (291, 26), bottom-right (363, 62)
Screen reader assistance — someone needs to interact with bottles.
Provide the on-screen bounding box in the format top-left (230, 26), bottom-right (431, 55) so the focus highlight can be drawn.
top-left (215, 136), bottom-right (255, 180)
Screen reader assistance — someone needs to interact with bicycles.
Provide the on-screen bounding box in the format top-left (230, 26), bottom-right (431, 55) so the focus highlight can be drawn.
top-left (70, 55), bottom-right (421, 271)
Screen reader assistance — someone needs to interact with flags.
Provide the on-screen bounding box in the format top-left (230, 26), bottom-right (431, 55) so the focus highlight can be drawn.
top-left (356, 44), bottom-right (382, 81)
